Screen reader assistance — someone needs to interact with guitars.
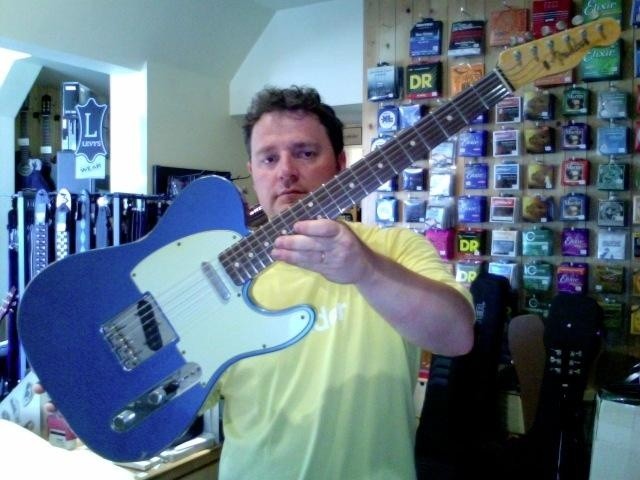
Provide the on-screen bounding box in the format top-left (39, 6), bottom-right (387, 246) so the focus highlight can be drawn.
top-left (16, 94), bottom-right (48, 214)
top-left (32, 96), bottom-right (55, 220)
top-left (17, 10), bottom-right (620, 462)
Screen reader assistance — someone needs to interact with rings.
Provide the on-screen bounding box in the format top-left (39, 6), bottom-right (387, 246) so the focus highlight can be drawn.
top-left (320, 251), bottom-right (326, 264)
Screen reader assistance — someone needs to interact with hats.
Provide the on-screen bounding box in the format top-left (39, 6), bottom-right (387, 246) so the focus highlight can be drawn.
top-left (469, 271), bottom-right (519, 364)
top-left (544, 291), bottom-right (604, 413)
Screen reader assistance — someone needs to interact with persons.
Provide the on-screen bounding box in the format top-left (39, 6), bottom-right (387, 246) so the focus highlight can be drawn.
top-left (34, 87), bottom-right (476, 480)
